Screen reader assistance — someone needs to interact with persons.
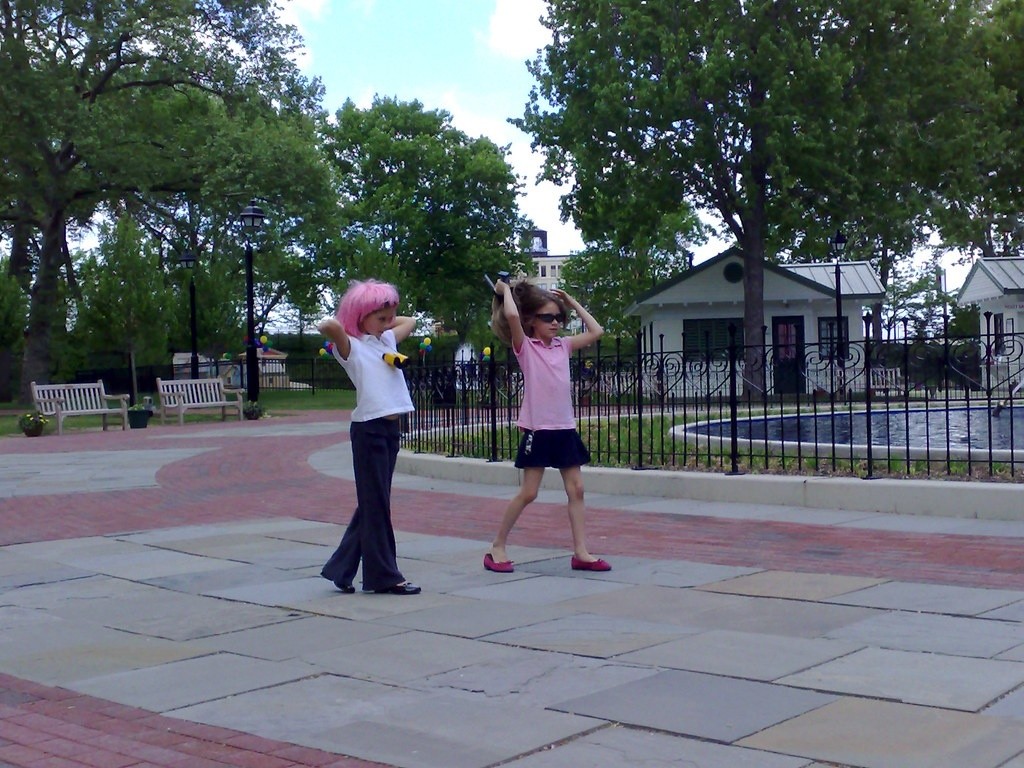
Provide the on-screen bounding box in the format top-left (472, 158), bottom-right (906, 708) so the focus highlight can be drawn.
top-left (316, 278), bottom-right (421, 595)
top-left (483, 278), bottom-right (611, 573)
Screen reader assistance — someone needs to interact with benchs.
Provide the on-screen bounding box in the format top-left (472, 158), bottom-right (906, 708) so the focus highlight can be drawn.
top-left (510, 372), bottom-right (578, 407)
top-left (31, 380), bottom-right (130, 436)
top-left (157, 375), bottom-right (245, 426)
top-left (836, 365), bottom-right (908, 401)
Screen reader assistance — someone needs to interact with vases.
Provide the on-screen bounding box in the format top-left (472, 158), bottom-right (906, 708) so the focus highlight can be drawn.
top-left (128, 411), bottom-right (153, 428)
top-left (244, 407), bottom-right (261, 419)
top-left (24, 424), bottom-right (43, 436)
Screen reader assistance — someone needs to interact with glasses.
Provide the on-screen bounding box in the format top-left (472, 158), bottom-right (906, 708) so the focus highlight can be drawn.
top-left (535, 314), bottom-right (565, 323)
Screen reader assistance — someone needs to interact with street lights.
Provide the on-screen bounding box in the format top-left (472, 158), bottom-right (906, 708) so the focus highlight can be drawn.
top-left (830, 228), bottom-right (849, 404)
top-left (177, 248), bottom-right (200, 379)
top-left (237, 197), bottom-right (265, 402)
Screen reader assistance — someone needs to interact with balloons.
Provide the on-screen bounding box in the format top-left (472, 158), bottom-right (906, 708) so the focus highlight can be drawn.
top-left (242, 334), bottom-right (273, 353)
top-left (318, 341), bottom-right (335, 356)
top-left (418, 338), bottom-right (433, 358)
top-left (479, 347), bottom-right (491, 361)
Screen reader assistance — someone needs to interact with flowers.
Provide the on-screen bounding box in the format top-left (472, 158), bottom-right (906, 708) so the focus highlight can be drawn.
top-left (129, 404), bottom-right (147, 412)
top-left (243, 400), bottom-right (265, 413)
top-left (19, 411), bottom-right (49, 430)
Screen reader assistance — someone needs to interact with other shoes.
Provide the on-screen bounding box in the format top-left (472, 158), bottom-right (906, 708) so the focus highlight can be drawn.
top-left (571, 556), bottom-right (612, 571)
top-left (374, 582), bottom-right (421, 595)
top-left (320, 572), bottom-right (355, 593)
top-left (484, 553), bottom-right (514, 572)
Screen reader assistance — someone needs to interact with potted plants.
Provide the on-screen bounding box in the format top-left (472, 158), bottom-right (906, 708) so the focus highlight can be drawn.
top-left (582, 390), bottom-right (593, 405)
